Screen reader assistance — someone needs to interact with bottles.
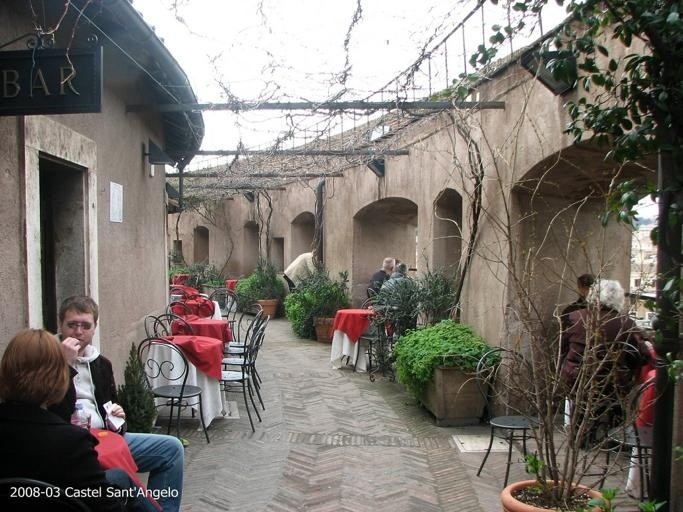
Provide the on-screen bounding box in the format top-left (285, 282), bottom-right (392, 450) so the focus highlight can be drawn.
top-left (70, 403), bottom-right (88, 430)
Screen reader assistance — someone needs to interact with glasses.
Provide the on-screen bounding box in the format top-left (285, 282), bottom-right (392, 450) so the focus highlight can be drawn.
top-left (65, 320), bottom-right (92, 329)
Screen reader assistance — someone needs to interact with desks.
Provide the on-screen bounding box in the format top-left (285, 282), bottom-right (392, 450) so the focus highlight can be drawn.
top-left (336, 307), bottom-right (395, 374)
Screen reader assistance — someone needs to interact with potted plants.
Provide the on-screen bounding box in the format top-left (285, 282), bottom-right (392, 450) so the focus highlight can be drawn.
top-left (202, 259), bottom-right (351, 343)
top-left (501, 450), bottom-right (611, 512)
top-left (392, 318), bottom-right (503, 427)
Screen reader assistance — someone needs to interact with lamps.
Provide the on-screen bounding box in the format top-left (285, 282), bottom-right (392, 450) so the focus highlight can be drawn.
top-left (143, 136), bottom-right (175, 165)
top-left (520, 37), bottom-right (577, 95)
top-left (367, 157), bottom-right (384, 177)
top-left (243, 190), bottom-right (254, 202)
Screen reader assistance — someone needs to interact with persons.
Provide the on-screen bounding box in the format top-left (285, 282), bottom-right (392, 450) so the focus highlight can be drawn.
top-left (367, 257), bottom-right (416, 337)
top-left (49, 297), bottom-right (183, 512)
top-left (0, 328), bottom-right (158, 512)
top-left (559, 273), bottom-right (652, 449)
top-left (284, 248), bottom-right (320, 293)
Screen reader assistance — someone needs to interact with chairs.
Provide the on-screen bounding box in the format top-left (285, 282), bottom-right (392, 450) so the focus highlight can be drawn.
top-left (596, 374), bottom-right (658, 511)
top-left (475, 346), bottom-right (554, 488)
top-left (0, 477), bottom-right (90, 512)
top-left (138, 272), bottom-right (270, 445)
